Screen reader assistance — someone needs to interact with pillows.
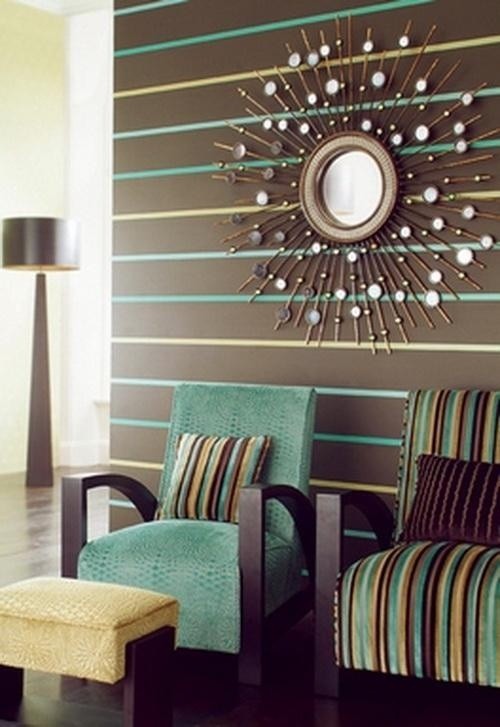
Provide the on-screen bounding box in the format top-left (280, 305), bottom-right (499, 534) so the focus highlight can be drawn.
top-left (398, 453), bottom-right (500, 545)
top-left (154, 432), bottom-right (270, 524)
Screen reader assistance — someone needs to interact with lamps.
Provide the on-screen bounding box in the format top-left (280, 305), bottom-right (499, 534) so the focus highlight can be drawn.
top-left (2, 216), bottom-right (81, 488)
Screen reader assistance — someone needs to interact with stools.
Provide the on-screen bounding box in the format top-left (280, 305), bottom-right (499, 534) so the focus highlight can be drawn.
top-left (0, 577), bottom-right (179, 727)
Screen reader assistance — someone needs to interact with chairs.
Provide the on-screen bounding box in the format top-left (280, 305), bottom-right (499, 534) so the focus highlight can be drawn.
top-left (313, 388), bottom-right (500, 700)
top-left (61, 382), bottom-right (317, 705)
